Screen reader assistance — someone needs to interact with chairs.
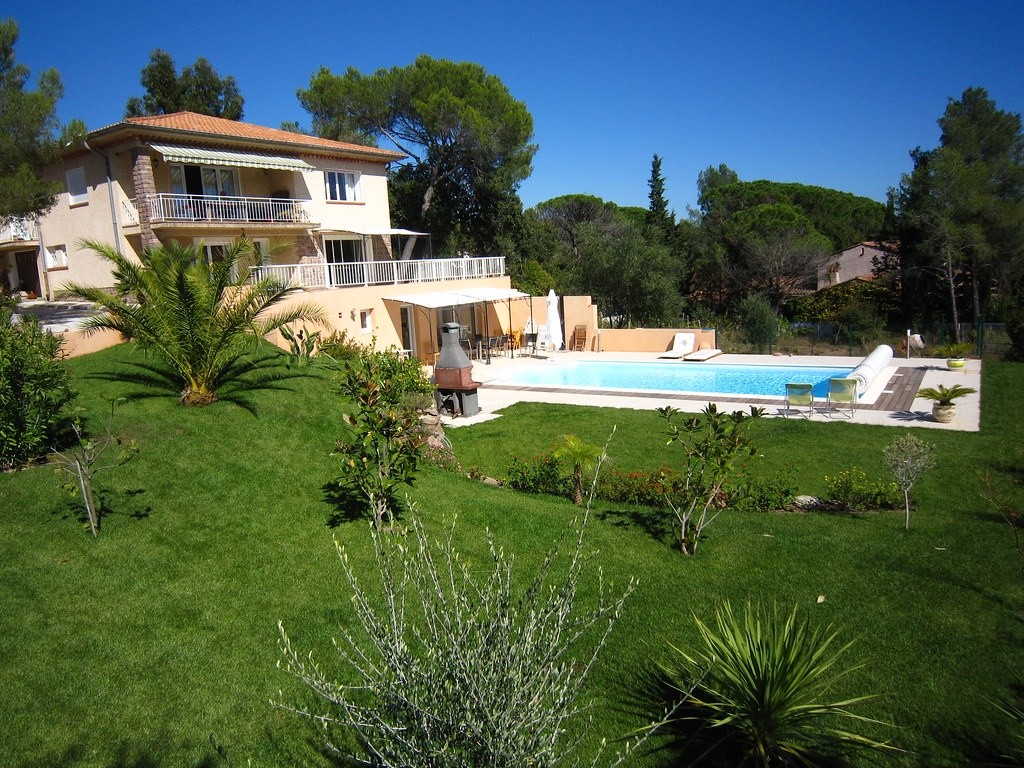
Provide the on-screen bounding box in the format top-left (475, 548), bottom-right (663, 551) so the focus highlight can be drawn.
top-left (684, 349), bottom-right (723, 362)
top-left (785, 383), bottom-right (815, 419)
top-left (572, 325), bottom-right (588, 352)
top-left (659, 333), bottom-right (695, 359)
top-left (827, 378), bottom-right (858, 420)
top-left (423, 340), bottom-right (440, 367)
top-left (460, 327), bottom-right (538, 360)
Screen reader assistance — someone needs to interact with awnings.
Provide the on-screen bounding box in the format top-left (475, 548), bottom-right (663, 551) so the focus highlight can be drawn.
top-left (381, 287), bottom-right (535, 365)
top-left (313, 226), bottom-right (432, 262)
top-left (139, 137), bottom-right (316, 173)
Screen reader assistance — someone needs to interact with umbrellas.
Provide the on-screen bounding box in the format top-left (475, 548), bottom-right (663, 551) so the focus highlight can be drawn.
top-left (545, 289), bottom-right (562, 352)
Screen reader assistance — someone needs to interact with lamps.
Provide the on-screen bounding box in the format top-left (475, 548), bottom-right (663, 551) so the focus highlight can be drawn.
top-left (264, 169), bottom-right (268, 176)
top-left (153, 158), bottom-right (159, 167)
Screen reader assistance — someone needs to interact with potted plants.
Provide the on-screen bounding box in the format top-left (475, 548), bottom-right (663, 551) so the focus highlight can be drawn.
top-left (914, 384), bottom-right (979, 423)
top-left (942, 342), bottom-right (977, 370)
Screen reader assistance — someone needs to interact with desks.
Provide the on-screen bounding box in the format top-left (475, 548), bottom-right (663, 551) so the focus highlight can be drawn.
top-left (392, 349), bottom-right (412, 363)
